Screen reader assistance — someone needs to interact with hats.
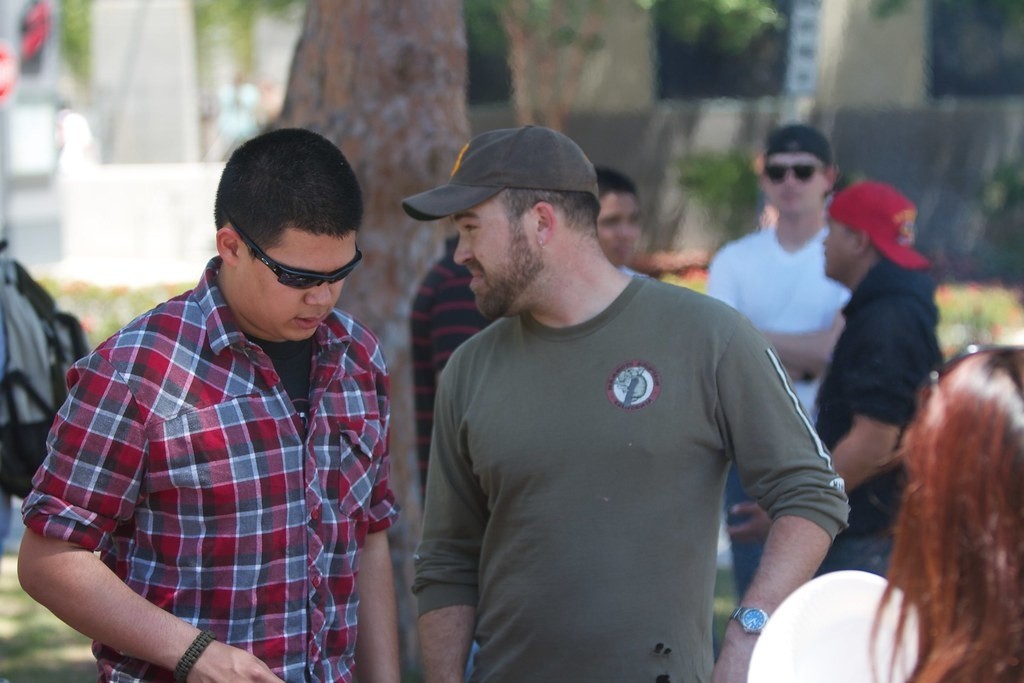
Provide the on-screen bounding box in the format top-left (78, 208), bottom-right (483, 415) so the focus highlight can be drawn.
top-left (765, 123), bottom-right (831, 163)
top-left (827, 181), bottom-right (931, 269)
top-left (401, 125), bottom-right (600, 221)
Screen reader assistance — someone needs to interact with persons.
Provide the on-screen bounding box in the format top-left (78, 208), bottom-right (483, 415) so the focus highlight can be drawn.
top-left (723, 181), bottom-right (945, 582)
top-left (17, 128), bottom-right (401, 683)
top-left (747, 346), bottom-right (1024, 683)
top-left (410, 238), bottom-right (493, 515)
top-left (592, 164), bottom-right (658, 280)
top-left (707, 124), bottom-right (851, 605)
top-left (413, 126), bottom-right (853, 683)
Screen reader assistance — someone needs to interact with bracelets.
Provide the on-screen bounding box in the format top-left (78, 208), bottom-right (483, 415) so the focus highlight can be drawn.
top-left (173, 629), bottom-right (217, 682)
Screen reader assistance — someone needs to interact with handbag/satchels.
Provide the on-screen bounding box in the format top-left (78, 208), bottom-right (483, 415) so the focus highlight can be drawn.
top-left (0, 370), bottom-right (59, 500)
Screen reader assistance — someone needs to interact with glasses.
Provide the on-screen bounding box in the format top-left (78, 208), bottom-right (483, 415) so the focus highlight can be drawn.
top-left (763, 163), bottom-right (828, 182)
top-left (227, 215), bottom-right (363, 289)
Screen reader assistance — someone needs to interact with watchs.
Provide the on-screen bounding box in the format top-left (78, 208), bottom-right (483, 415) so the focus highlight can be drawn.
top-left (729, 607), bottom-right (769, 635)
